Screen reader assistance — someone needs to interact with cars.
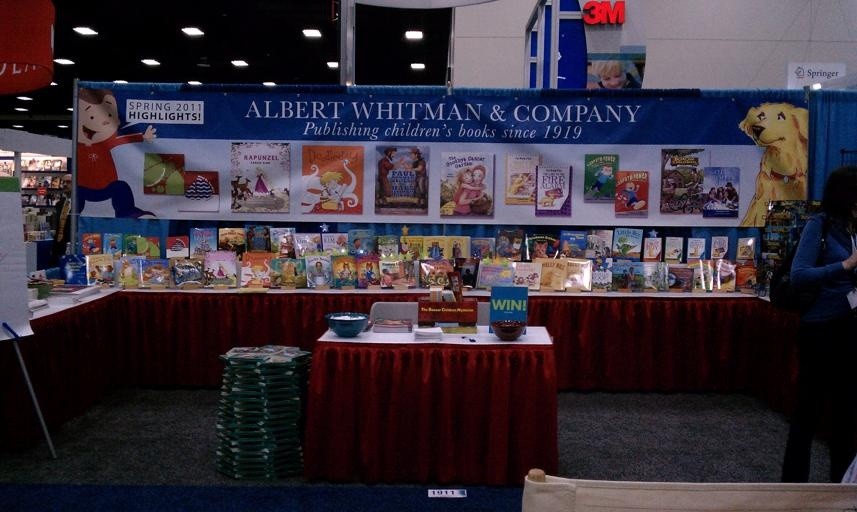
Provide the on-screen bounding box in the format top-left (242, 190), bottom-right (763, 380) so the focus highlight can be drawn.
top-left (662, 186), bottom-right (689, 212)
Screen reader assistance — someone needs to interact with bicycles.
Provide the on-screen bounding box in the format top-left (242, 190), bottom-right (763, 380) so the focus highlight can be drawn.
top-left (681, 183), bottom-right (702, 214)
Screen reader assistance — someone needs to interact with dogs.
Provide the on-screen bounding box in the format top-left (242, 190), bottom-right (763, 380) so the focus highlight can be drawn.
top-left (737, 102), bottom-right (809, 228)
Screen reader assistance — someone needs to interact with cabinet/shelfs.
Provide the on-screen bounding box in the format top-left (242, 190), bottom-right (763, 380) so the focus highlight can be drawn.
top-left (0, 149), bottom-right (72, 272)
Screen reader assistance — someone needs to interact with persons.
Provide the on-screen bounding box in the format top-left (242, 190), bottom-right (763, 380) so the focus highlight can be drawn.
top-left (582, 61), bottom-right (639, 91)
top-left (452, 168), bottom-right (487, 204)
top-left (590, 164), bottom-right (614, 193)
top-left (75, 86), bottom-right (158, 220)
top-left (615, 181), bottom-right (646, 210)
top-left (253, 165), bottom-right (270, 198)
top-left (377, 147), bottom-right (403, 204)
top-left (452, 164), bottom-right (486, 215)
top-left (400, 148), bottom-right (427, 209)
top-left (48, 173), bottom-right (71, 267)
top-left (777, 166), bottom-right (855, 483)
top-left (320, 171), bottom-right (343, 211)
top-left (703, 182), bottom-right (737, 212)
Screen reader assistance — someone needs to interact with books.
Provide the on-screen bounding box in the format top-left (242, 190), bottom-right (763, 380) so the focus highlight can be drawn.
top-left (488, 286), bottom-right (528, 335)
top-left (214, 344), bottom-right (312, 480)
top-left (58, 228), bottom-right (760, 294)
top-left (372, 318), bottom-right (412, 333)
top-left (413, 326), bottom-right (443, 340)
top-left (0, 156), bottom-right (62, 242)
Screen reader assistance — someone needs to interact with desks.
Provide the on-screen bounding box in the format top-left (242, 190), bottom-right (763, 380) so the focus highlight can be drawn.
top-left (1, 287), bottom-right (780, 488)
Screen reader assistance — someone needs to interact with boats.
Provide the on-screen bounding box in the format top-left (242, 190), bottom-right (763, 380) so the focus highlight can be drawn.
top-left (703, 199), bottom-right (738, 217)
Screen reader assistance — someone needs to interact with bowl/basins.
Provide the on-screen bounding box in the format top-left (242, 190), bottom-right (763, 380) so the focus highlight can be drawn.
top-left (323, 312), bottom-right (368, 337)
top-left (490, 321), bottom-right (527, 342)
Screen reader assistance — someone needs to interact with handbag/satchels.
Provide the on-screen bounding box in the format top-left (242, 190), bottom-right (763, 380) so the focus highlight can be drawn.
top-left (769, 215), bottom-right (829, 312)
